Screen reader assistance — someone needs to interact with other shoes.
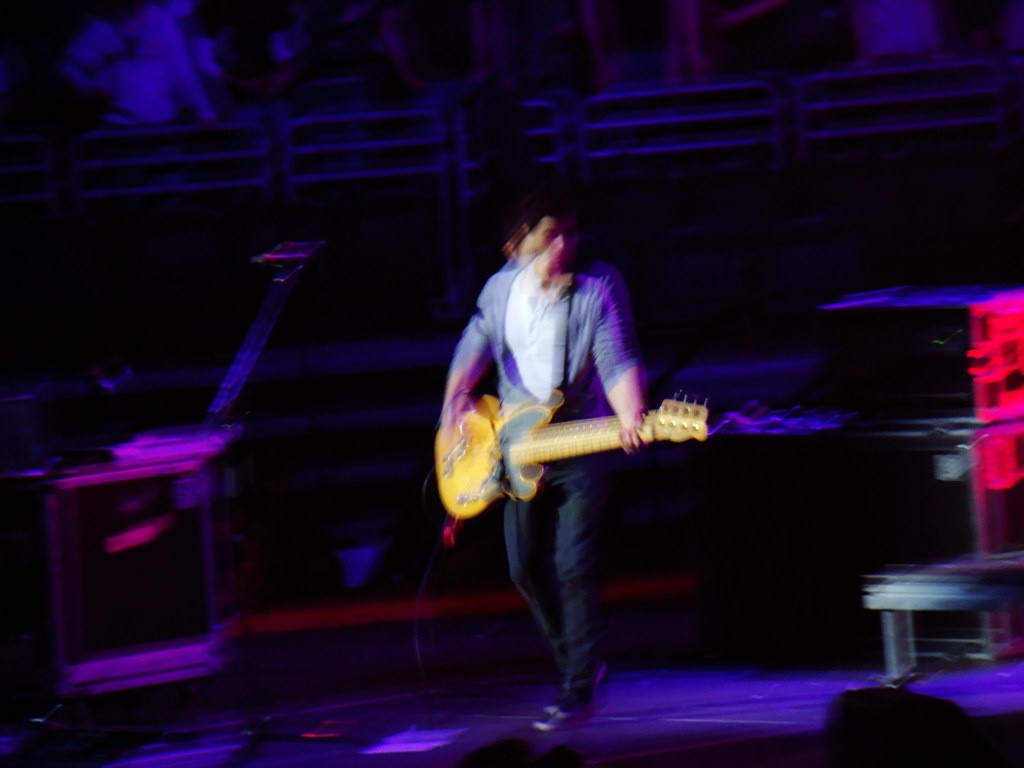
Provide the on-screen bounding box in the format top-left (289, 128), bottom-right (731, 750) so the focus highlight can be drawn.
top-left (533, 700), bottom-right (595, 731)
top-left (544, 660), bottom-right (607, 715)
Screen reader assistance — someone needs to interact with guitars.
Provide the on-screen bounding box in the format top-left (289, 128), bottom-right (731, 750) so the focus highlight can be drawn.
top-left (432, 389), bottom-right (711, 521)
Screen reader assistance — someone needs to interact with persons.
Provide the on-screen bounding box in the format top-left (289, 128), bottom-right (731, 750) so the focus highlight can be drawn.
top-left (436, 192), bottom-right (646, 732)
top-left (0, 0), bottom-right (1024, 228)
